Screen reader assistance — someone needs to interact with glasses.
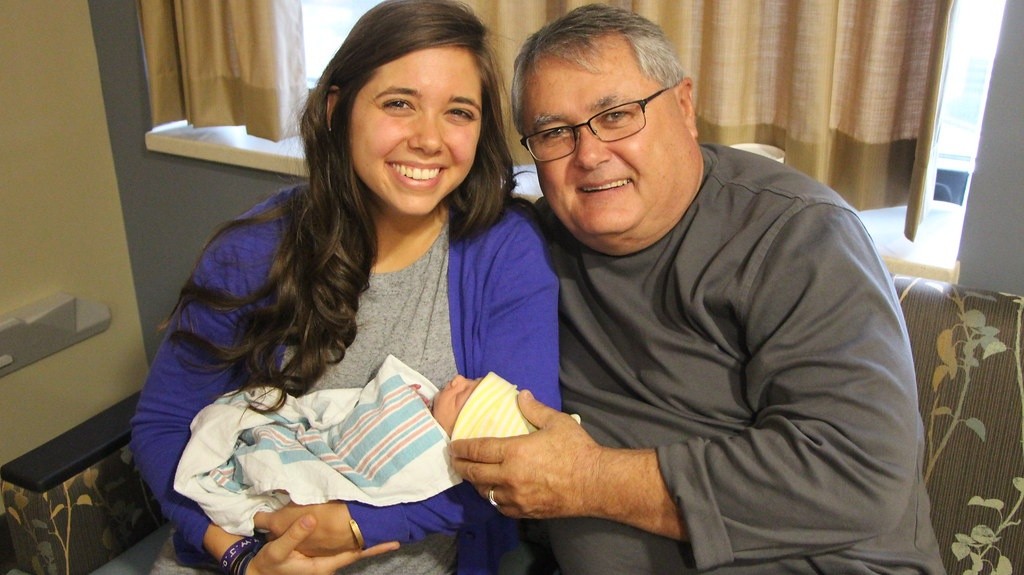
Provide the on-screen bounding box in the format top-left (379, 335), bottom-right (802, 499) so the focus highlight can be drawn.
top-left (520, 88), bottom-right (671, 161)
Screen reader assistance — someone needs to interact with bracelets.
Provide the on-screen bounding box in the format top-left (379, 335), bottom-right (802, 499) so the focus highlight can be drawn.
top-left (349, 518), bottom-right (366, 548)
top-left (220, 537), bottom-right (268, 575)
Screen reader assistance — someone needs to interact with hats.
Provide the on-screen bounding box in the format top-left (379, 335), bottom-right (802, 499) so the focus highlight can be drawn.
top-left (450, 372), bottom-right (581, 442)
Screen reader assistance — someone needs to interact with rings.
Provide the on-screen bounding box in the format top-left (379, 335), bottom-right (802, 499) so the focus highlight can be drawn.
top-left (488, 486), bottom-right (499, 510)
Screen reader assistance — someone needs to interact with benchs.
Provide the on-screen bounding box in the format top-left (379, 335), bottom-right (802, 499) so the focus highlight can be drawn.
top-left (0, 271), bottom-right (1024, 575)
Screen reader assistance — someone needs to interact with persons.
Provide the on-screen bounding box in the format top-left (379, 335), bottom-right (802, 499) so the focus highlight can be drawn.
top-left (496, 3), bottom-right (947, 574)
top-left (88, 0), bottom-right (563, 574)
top-left (173, 353), bottom-right (582, 536)
